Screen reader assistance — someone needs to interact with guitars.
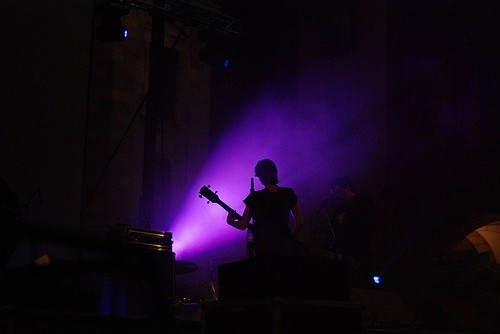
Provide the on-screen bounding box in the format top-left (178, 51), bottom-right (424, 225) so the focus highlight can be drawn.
top-left (197, 184), bottom-right (310, 268)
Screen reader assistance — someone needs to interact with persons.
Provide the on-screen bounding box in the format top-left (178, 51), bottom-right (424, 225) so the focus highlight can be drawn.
top-left (227, 159), bottom-right (310, 260)
top-left (442, 196), bottom-right (500, 271)
top-left (328, 176), bottom-right (375, 250)
